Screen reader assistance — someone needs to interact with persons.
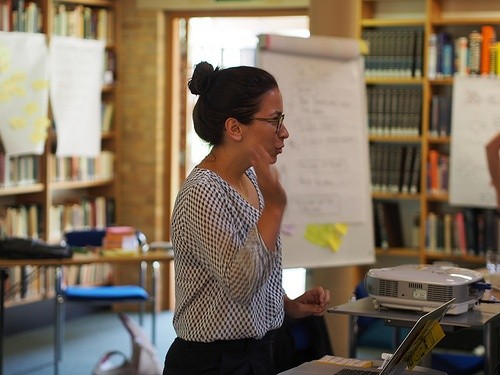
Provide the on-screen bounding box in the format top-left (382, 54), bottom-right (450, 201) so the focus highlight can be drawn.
top-left (162, 62), bottom-right (331, 375)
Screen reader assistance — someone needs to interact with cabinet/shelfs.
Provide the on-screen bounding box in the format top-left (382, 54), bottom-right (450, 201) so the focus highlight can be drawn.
top-left (350, 0), bottom-right (500, 266)
top-left (0, 0), bottom-right (122, 310)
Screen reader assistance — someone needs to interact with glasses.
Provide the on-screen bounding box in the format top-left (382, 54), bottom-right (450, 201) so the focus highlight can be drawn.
top-left (224, 114), bottom-right (286, 134)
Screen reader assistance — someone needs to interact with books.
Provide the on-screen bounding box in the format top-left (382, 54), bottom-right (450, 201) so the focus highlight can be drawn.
top-left (0, 0), bottom-right (115, 84)
top-left (0, 104), bottom-right (114, 189)
top-left (374, 201), bottom-right (500, 260)
top-left (361, 26), bottom-right (500, 81)
top-left (0, 195), bottom-right (136, 303)
top-left (366, 88), bottom-right (452, 138)
top-left (369, 144), bottom-right (449, 196)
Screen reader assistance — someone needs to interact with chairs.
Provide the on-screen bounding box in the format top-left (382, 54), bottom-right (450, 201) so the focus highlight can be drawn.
top-left (55, 230), bottom-right (160, 375)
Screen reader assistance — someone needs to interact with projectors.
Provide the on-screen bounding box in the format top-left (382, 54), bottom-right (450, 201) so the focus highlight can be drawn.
top-left (365, 265), bottom-right (485, 315)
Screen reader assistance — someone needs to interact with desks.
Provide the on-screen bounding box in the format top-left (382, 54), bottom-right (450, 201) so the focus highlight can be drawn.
top-left (0, 244), bottom-right (173, 374)
top-left (326, 268), bottom-right (500, 375)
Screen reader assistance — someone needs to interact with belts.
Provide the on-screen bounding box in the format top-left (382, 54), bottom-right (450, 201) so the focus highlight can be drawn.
top-left (176, 335), bottom-right (268, 346)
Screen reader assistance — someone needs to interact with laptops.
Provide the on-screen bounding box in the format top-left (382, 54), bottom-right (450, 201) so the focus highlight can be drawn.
top-left (275, 298), bottom-right (456, 375)
